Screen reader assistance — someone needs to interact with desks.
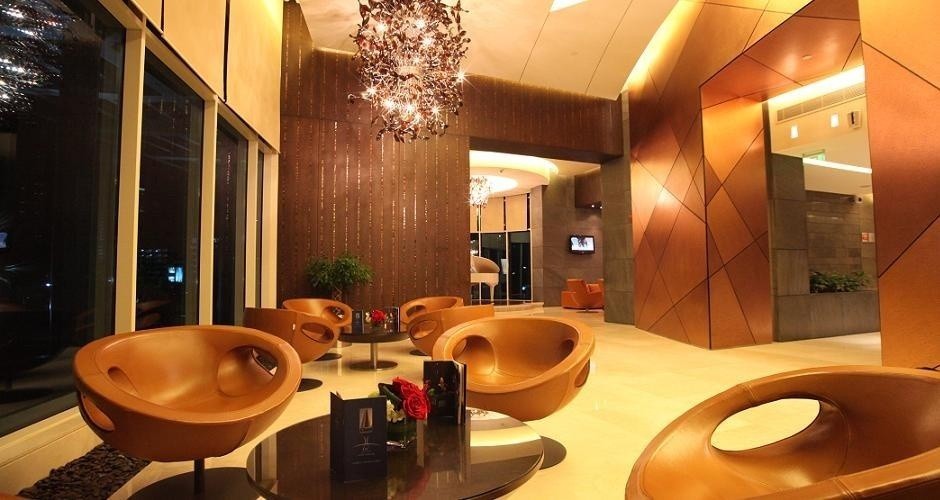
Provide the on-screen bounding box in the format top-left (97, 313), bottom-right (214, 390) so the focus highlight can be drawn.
top-left (337, 321), bottom-right (410, 372)
top-left (245, 406), bottom-right (545, 499)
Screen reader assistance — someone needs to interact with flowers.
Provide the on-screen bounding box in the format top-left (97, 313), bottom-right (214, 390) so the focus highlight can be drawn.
top-left (367, 377), bottom-right (435, 423)
top-left (371, 308), bottom-right (388, 324)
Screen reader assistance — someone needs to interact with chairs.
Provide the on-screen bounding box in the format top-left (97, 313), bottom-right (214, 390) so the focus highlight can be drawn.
top-left (72, 326), bottom-right (303, 500)
top-left (406, 304), bottom-right (494, 360)
top-left (625, 365), bottom-right (940, 499)
top-left (242, 307), bottom-right (341, 392)
top-left (399, 296), bottom-right (465, 357)
top-left (432, 315), bottom-right (596, 469)
top-left (596, 278), bottom-right (605, 307)
top-left (567, 279), bottom-right (603, 313)
top-left (282, 298), bottom-right (354, 362)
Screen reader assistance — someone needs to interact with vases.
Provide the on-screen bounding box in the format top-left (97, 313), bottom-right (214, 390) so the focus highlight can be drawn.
top-left (378, 383), bottom-right (418, 447)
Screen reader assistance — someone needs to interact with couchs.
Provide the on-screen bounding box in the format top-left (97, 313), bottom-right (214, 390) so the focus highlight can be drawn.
top-left (561, 283), bottom-right (601, 309)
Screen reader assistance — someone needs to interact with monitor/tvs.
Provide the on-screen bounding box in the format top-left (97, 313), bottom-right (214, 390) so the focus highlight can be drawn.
top-left (569, 236), bottom-right (595, 254)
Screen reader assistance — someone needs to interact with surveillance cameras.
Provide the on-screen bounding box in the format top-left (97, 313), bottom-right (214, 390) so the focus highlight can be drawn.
top-left (855, 194), bottom-right (864, 203)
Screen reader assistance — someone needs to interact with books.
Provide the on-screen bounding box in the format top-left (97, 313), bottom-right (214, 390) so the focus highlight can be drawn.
top-left (385, 306), bottom-right (400, 333)
top-left (352, 309), bottom-right (364, 335)
top-left (423, 360), bottom-right (467, 426)
top-left (330, 391), bottom-right (388, 484)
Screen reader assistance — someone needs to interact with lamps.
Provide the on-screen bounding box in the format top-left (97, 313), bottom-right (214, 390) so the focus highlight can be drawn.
top-left (347, 0), bottom-right (469, 144)
top-left (469, 177), bottom-right (493, 207)
top-left (1, 0), bottom-right (70, 112)
